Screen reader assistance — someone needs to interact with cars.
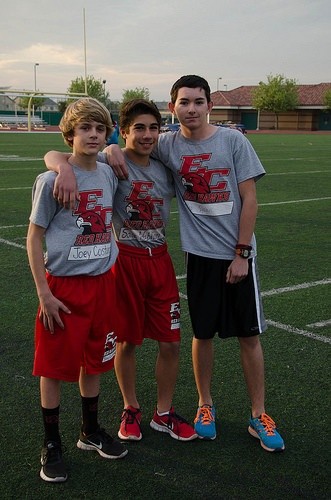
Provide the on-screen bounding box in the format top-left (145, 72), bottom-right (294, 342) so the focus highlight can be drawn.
top-left (160, 123), bottom-right (181, 133)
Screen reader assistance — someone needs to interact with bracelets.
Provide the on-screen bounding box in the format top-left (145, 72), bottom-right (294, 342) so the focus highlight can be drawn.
top-left (236, 243), bottom-right (253, 251)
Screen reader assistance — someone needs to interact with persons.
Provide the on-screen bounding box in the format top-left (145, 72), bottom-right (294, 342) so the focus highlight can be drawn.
top-left (42, 98), bottom-right (199, 442)
top-left (102, 75), bottom-right (285, 452)
top-left (25, 97), bottom-right (127, 481)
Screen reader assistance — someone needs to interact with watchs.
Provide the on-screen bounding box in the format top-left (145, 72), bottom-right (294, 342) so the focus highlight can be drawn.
top-left (234, 249), bottom-right (251, 259)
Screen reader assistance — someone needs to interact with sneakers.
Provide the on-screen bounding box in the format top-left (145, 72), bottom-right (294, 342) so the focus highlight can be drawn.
top-left (77, 426), bottom-right (129, 459)
top-left (117, 405), bottom-right (143, 441)
top-left (149, 404), bottom-right (198, 440)
top-left (248, 411), bottom-right (286, 452)
top-left (193, 403), bottom-right (217, 440)
top-left (40, 442), bottom-right (68, 483)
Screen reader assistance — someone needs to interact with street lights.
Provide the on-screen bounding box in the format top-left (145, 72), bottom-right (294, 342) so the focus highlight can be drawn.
top-left (217, 77), bottom-right (222, 90)
top-left (224, 85), bottom-right (227, 91)
top-left (103, 79), bottom-right (106, 97)
top-left (34, 63), bottom-right (39, 91)
